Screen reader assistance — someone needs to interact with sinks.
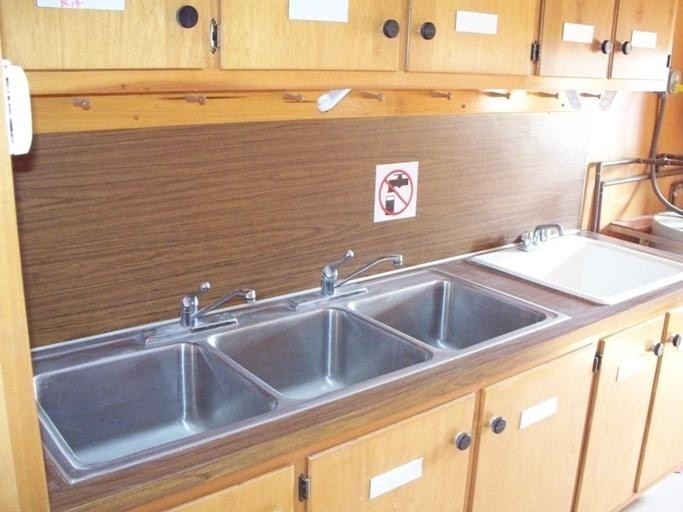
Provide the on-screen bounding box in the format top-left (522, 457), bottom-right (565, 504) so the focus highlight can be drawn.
top-left (31, 333), bottom-right (283, 486)
top-left (204, 303), bottom-right (437, 404)
top-left (347, 267), bottom-right (571, 354)
top-left (464, 225), bottom-right (683, 308)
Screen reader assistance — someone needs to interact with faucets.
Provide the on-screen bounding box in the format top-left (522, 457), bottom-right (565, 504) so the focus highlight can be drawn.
top-left (178, 279), bottom-right (257, 331)
top-left (530, 223), bottom-right (564, 245)
top-left (321, 248), bottom-right (405, 297)
